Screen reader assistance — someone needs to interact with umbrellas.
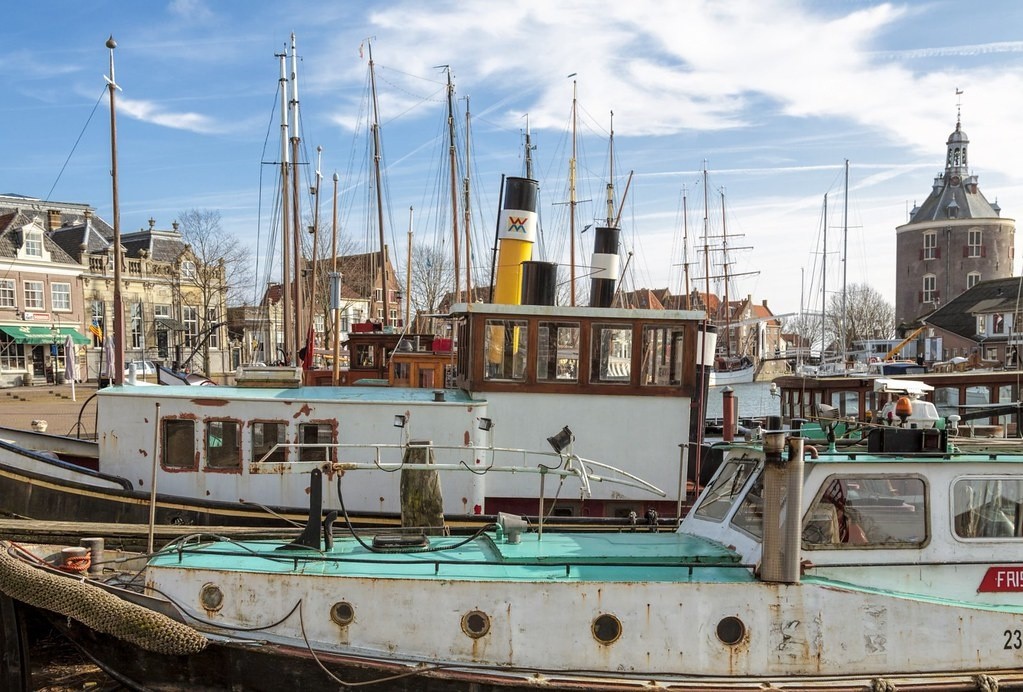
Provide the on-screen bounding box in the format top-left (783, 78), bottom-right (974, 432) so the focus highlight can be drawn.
top-left (105, 336), bottom-right (115, 387)
top-left (64, 334), bottom-right (76, 402)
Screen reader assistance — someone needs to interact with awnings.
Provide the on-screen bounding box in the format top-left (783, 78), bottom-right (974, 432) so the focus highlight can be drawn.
top-left (154, 319), bottom-right (192, 331)
top-left (0, 325), bottom-right (91, 345)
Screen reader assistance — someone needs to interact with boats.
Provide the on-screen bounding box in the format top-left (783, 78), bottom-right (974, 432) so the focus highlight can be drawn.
top-left (0, 28), bottom-right (1023, 692)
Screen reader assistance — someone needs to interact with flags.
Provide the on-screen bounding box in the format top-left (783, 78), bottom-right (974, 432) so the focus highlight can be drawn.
top-left (995, 313), bottom-right (1002, 333)
top-left (88, 319), bottom-right (102, 343)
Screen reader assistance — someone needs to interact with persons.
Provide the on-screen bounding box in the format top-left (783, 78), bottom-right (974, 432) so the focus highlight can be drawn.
top-left (893, 353), bottom-right (900, 360)
top-left (917, 353), bottom-right (925, 365)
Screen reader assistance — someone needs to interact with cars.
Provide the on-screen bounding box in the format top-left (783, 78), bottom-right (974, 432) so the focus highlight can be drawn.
top-left (124, 360), bottom-right (156, 374)
